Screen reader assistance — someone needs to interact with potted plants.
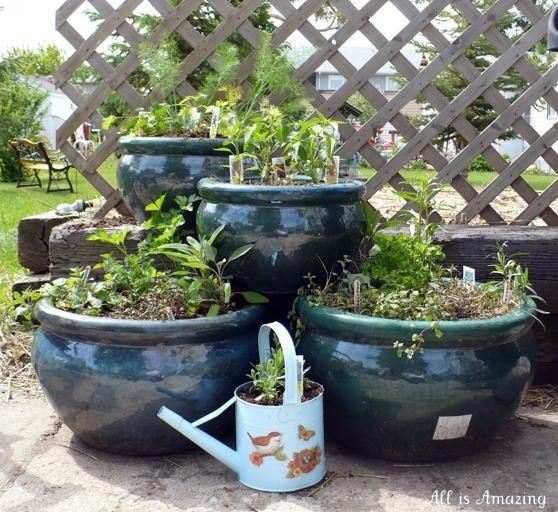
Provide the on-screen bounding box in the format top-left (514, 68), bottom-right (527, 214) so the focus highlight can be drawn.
top-left (116, 103), bottom-right (234, 222)
top-left (157, 322), bottom-right (325, 493)
top-left (32, 192), bottom-right (260, 457)
top-left (198, 123), bottom-right (369, 294)
top-left (295, 158), bottom-right (538, 467)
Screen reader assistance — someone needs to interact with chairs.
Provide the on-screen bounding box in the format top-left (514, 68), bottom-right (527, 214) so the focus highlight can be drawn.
top-left (8, 139), bottom-right (74, 193)
